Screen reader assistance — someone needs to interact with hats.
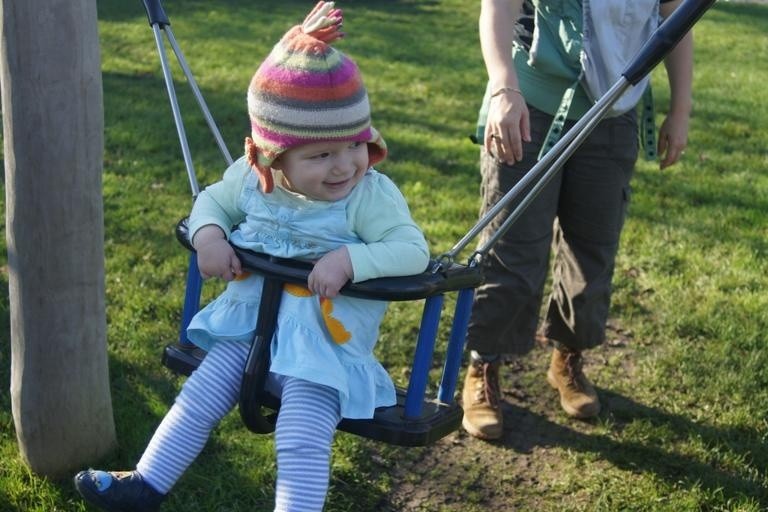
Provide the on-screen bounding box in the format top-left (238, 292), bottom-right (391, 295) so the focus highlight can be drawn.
top-left (245, 1), bottom-right (387, 194)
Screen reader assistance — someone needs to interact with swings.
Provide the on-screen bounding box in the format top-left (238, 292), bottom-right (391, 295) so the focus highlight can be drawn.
top-left (143, 0), bottom-right (715, 446)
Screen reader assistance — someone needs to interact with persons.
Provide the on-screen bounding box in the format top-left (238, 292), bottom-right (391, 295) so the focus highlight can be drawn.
top-left (75, 1), bottom-right (431, 512)
top-left (461, 0), bottom-right (694, 441)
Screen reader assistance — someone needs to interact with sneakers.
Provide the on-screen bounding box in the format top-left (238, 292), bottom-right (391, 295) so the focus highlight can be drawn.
top-left (74, 470), bottom-right (169, 512)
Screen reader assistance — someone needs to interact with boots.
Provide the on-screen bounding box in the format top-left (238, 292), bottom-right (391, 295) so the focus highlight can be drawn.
top-left (546, 346), bottom-right (601, 417)
top-left (462, 350), bottom-right (504, 440)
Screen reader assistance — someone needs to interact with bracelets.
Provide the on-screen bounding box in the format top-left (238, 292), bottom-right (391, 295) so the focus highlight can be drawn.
top-left (490, 86), bottom-right (522, 97)
